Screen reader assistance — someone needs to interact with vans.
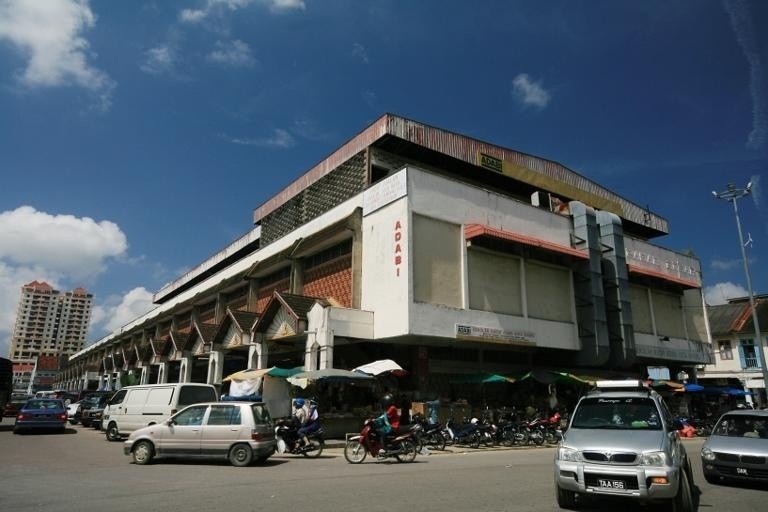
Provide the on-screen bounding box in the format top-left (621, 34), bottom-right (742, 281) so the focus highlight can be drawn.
top-left (101, 382), bottom-right (219, 441)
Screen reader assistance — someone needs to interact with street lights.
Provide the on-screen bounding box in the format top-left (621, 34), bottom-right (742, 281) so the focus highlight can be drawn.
top-left (710, 179), bottom-right (767, 400)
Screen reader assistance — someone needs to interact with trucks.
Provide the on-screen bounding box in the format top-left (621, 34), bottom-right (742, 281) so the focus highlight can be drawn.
top-left (0, 357), bottom-right (12, 423)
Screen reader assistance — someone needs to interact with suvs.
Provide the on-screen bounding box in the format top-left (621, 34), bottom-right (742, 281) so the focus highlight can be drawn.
top-left (552, 380), bottom-right (695, 512)
top-left (121, 401), bottom-right (277, 469)
top-left (34, 390), bottom-right (72, 406)
top-left (74, 391), bottom-right (118, 428)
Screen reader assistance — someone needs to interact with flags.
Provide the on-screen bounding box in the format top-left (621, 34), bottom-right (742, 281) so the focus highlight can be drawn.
top-left (548, 192), bottom-right (571, 217)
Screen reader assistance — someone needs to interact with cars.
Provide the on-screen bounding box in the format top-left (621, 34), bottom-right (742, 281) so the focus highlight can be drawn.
top-left (4, 392), bottom-right (36, 417)
top-left (13, 398), bottom-right (67, 435)
top-left (699, 410), bottom-right (767, 487)
top-left (81, 396), bottom-right (112, 423)
top-left (65, 400), bottom-right (83, 425)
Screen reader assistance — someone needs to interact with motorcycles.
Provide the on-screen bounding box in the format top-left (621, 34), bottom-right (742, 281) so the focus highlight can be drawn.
top-left (274, 416), bottom-right (327, 458)
top-left (343, 416), bottom-right (423, 464)
top-left (677, 412), bottom-right (713, 435)
top-left (409, 405), bottom-right (565, 453)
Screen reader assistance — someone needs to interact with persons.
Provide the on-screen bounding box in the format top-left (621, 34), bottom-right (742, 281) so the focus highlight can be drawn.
top-left (370, 398), bottom-right (392, 460)
top-left (680, 419), bottom-right (697, 438)
top-left (727, 400), bottom-right (759, 411)
top-left (623, 404), bottom-right (652, 427)
top-left (549, 406), bottom-right (561, 423)
top-left (296, 396), bottom-right (320, 450)
top-left (372, 393), bottom-right (402, 455)
top-left (273, 398), bottom-right (306, 453)
